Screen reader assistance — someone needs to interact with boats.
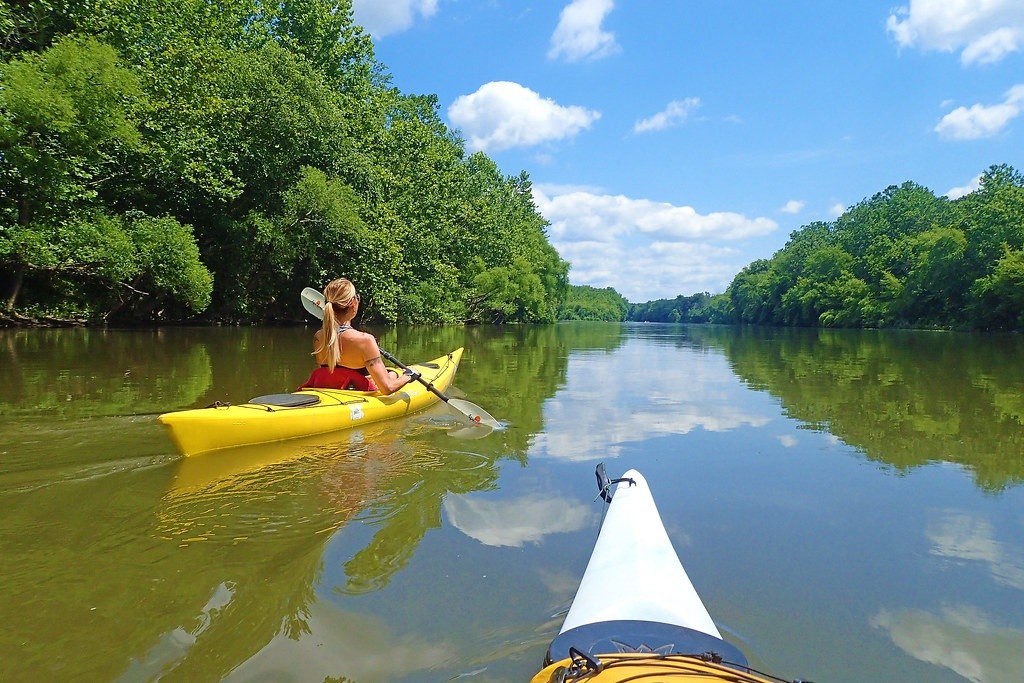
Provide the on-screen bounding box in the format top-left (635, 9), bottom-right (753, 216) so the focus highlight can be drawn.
top-left (156, 347), bottom-right (464, 457)
top-left (528, 463), bottom-right (814, 683)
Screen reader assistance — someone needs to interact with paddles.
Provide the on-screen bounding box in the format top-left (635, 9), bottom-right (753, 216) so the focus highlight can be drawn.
top-left (300, 286), bottom-right (503, 431)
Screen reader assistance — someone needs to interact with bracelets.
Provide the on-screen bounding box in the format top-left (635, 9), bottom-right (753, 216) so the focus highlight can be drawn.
top-left (402, 368), bottom-right (419, 383)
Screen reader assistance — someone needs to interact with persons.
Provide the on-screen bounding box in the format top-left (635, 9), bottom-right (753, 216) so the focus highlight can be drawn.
top-left (313, 277), bottom-right (420, 396)
